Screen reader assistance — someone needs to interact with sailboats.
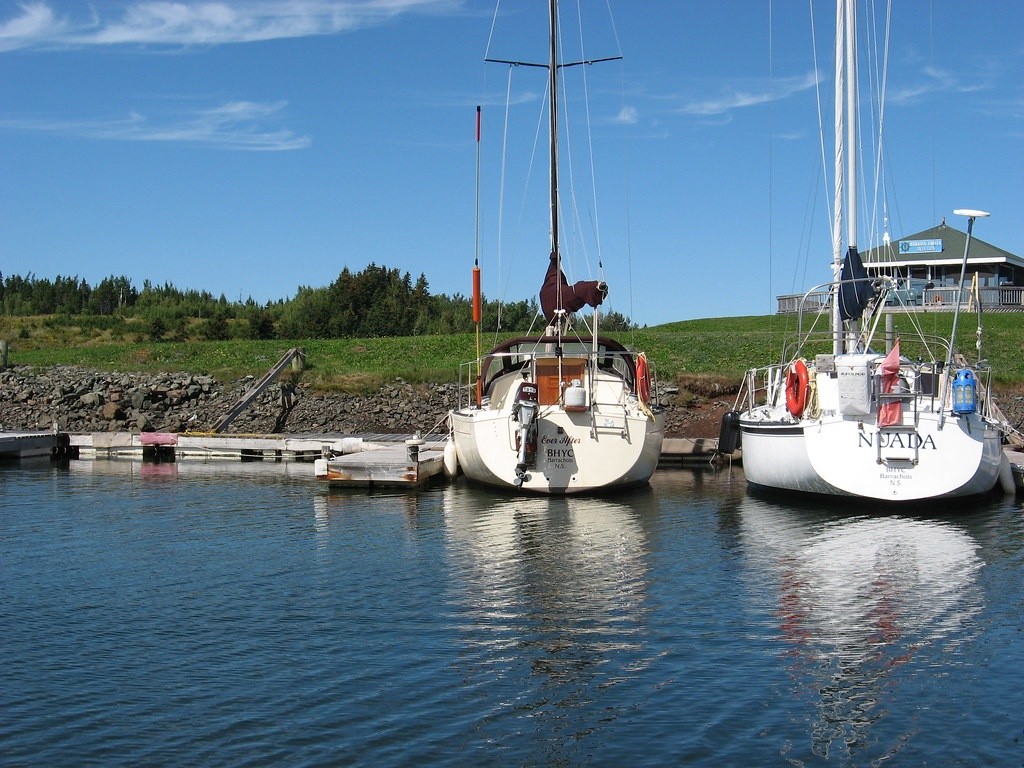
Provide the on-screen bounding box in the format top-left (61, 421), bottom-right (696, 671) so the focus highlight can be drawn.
top-left (453, 0), bottom-right (678, 496)
top-left (718, 1), bottom-right (1024, 506)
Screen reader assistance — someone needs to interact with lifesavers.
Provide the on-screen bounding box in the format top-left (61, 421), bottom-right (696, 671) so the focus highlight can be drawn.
top-left (637, 352), bottom-right (652, 401)
top-left (786, 360), bottom-right (810, 416)
top-left (717, 411), bottom-right (738, 454)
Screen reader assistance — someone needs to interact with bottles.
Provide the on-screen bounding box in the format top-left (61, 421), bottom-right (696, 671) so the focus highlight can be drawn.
top-left (53, 420), bottom-right (58, 433)
